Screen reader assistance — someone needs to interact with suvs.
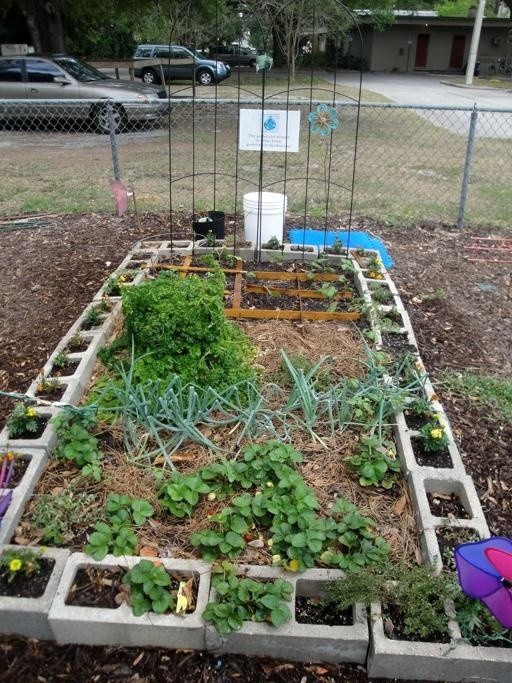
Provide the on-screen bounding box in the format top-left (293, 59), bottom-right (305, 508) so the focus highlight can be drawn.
top-left (207, 45), bottom-right (260, 67)
top-left (129, 43), bottom-right (231, 87)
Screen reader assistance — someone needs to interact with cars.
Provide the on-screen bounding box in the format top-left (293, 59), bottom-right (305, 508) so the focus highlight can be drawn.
top-left (0, 51), bottom-right (171, 132)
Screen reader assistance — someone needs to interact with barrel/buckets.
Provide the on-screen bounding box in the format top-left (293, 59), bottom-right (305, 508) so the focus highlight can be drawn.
top-left (242, 192), bottom-right (288, 242)
top-left (192, 211), bottom-right (225, 240)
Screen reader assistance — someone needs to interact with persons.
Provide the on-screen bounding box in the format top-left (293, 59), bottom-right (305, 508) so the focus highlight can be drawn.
top-left (265, 117), bottom-right (275, 130)
